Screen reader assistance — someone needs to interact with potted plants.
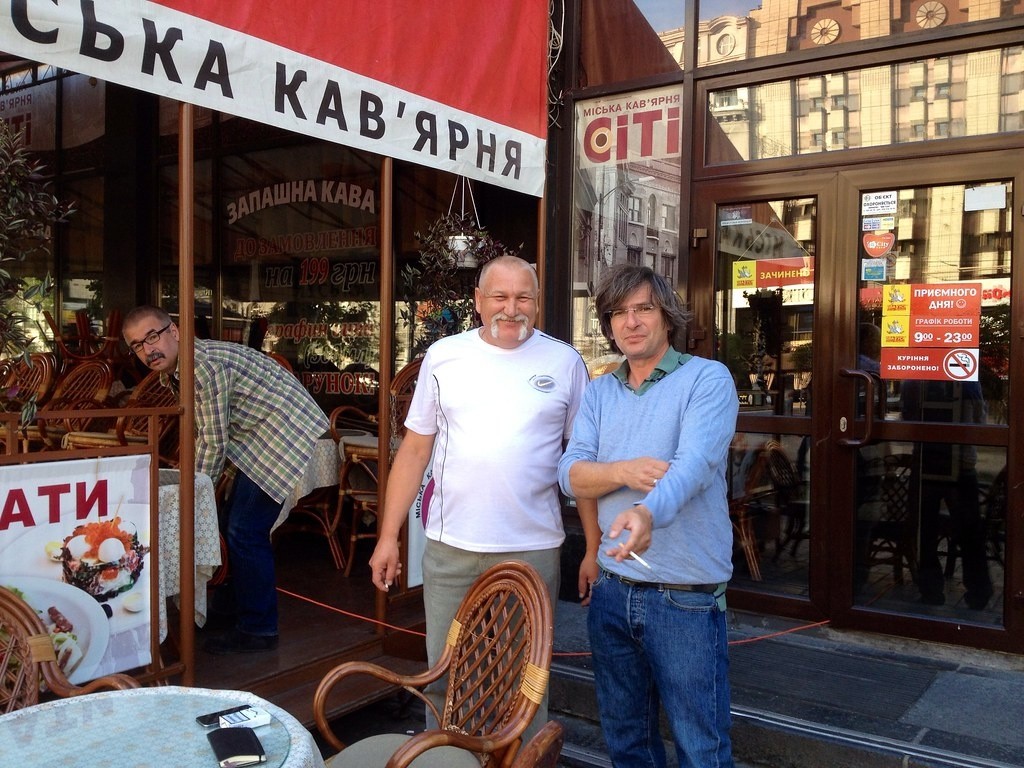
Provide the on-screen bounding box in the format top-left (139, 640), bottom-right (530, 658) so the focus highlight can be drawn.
top-left (401, 213), bottom-right (525, 283)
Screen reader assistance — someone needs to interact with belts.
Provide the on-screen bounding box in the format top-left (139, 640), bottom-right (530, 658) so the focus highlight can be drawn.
top-left (619, 575), bottom-right (718, 593)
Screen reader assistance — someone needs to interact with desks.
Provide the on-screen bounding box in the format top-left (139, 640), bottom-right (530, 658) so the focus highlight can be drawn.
top-left (0, 685), bottom-right (326, 768)
top-left (158, 468), bottom-right (222, 644)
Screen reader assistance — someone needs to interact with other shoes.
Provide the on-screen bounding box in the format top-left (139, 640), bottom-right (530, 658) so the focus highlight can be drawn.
top-left (205, 629), bottom-right (280, 656)
top-left (195, 602), bottom-right (241, 632)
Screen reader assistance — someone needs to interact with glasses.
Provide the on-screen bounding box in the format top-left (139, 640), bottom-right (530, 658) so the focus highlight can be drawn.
top-left (128, 324), bottom-right (170, 354)
top-left (609, 304), bottom-right (657, 319)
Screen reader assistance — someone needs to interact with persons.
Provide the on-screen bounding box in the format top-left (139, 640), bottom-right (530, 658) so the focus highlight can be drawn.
top-left (556, 261), bottom-right (737, 768)
top-left (795, 379), bottom-right (811, 472)
top-left (889, 376), bottom-right (994, 606)
top-left (366, 254), bottom-right (604, 763)
top-left (122, 306), bottom-right (332, 653)
top-left (855, 325), bottom-right (884, 605)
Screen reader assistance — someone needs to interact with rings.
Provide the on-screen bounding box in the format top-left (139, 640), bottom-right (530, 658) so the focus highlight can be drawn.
top-left (653, 479), bottom-right (657, 485)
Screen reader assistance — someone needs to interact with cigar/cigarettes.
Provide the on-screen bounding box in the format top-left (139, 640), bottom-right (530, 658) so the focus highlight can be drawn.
top-left (619, 543), bottom-right (652, 570)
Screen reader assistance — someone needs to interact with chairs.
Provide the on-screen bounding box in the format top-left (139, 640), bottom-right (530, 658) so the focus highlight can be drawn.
top-left (325, 357), bottom-right (424, 538)
top-left (729, 440), bottom-right (797, 582)
top-left (43, 309), bottom-right (123, 390)
top-left (0, 352), bottom-right (53, 454)
top-left (343, 460), bottom-right (379, 577)
top-left (312, 557), bottom-right (564, 768)
top-left (64, 369), bottom-right (180, 451)
top-left (854, 453), bottom-right (914, 595)
top-left (0, 585), bottom-right (144, 714)
top-left (38, 359), bottom-right (111, 447)
top-left (938, 464), bottom-right (1007, 595)
top-left (765, 440), bottom-right (810, 556)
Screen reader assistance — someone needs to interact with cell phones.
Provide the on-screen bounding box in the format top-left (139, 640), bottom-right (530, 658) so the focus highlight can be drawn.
top-left (196, 705), bottom-right (252, 728)
